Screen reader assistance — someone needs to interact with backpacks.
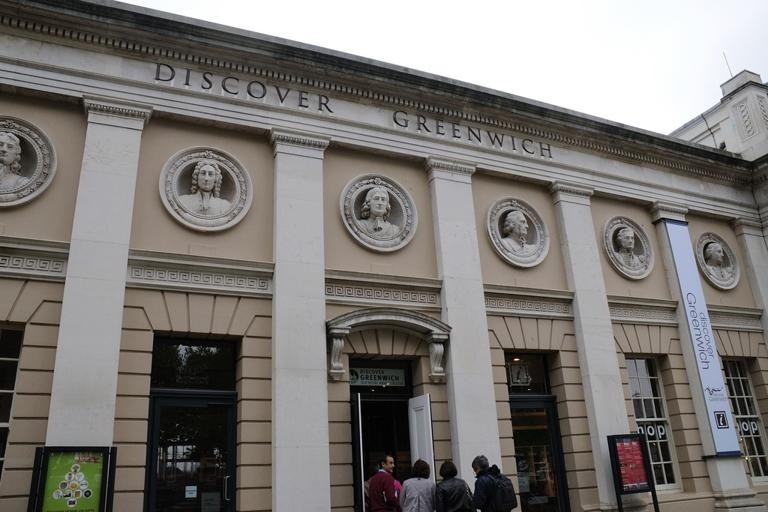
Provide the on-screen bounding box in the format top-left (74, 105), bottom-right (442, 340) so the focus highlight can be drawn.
top-left (478, 473), bottom-right (518, 512)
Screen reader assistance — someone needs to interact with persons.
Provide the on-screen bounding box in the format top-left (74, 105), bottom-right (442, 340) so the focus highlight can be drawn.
top-left (356, 185), bottom-right (404, 239)
top-left (0, 131), bottom-right (29, 193)
top-left (180, 160), bottom-right (233, 218)
top-left (613, 227), bottom-right (646, 267)
top-left (365, 455), bottom-right (518, 512)
top-left (705, 242), bottom-right (732, 280)
top-left (502, 208), bottom-right (540, 254)
